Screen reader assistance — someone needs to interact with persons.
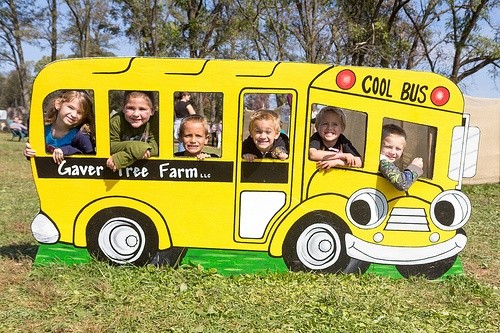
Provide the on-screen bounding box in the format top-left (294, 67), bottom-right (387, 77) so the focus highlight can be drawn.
top-left (309, 106), bottom-right (364, 172)
top-left (175, 91), bottom-right (196, 152)
top-left (174, 114), bottom-right (219, 160)
top-left (107, 91), bottom-right (158, 172)
top-left (10, 117), bottom-right (23, 142)
top-left (24, 89), bottom-right (95, 163)
top-left (380, 124), bottom-right (423, 191)
top-left (241, 109), bottom-right (289, 162)
top-left (211, 120), bottom-right (222, 148)
top-left (6, 110), bottom-right (14, 125)
top-left (18, 111), bottom-right (24, 124)
